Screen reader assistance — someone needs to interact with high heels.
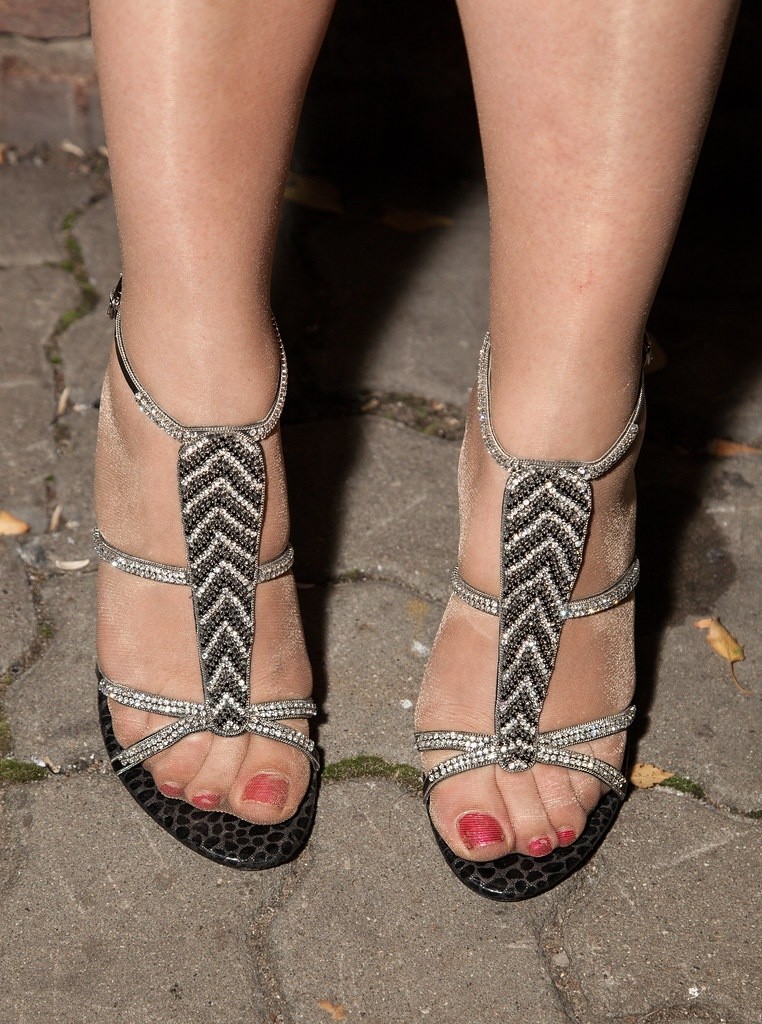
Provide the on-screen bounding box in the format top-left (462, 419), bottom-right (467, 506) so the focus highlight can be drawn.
top-left (413, 328), bottom-right (645, 903)
top-left (95, 273), bottom-right (322, 870)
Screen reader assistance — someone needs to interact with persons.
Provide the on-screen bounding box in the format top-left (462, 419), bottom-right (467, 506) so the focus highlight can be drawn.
top-left (86, 0), bottom-right (744, 899)
